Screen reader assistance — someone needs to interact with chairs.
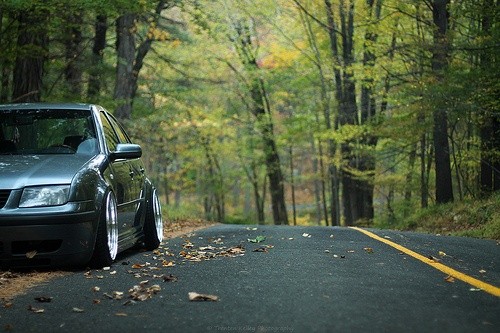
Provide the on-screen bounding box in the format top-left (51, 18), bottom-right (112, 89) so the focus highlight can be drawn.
top-left (0, 140), bottom-right (20, 155)
top-left (62, 135), bottom-right (98, 154)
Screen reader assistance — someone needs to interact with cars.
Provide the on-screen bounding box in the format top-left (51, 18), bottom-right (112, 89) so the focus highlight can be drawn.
top-left (0, 103), bottom-right (164, 267)
